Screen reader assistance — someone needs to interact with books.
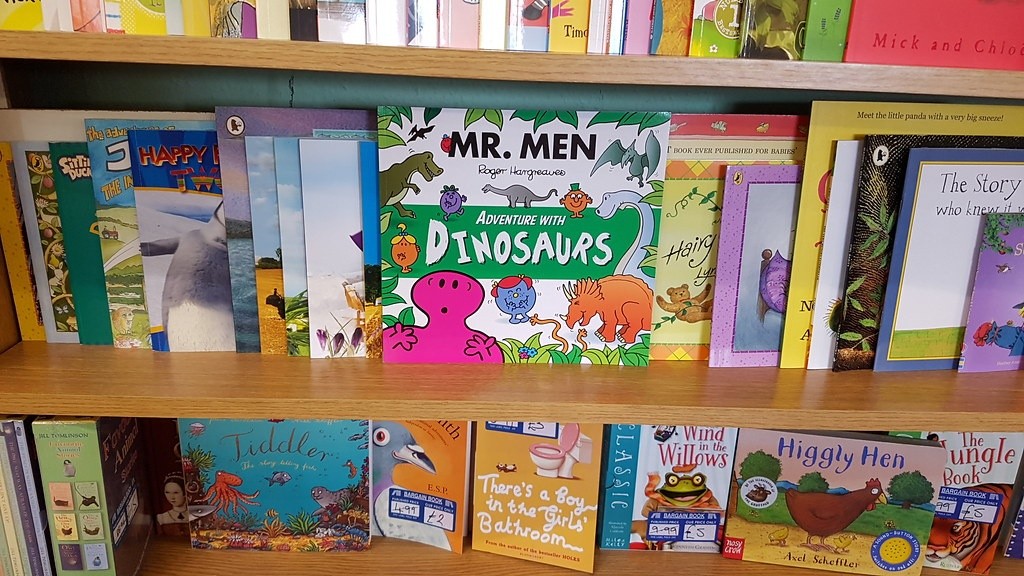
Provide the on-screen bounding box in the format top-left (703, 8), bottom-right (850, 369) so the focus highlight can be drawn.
top-left (0, 0), bottom-right (1024, 73)
top-left (0, 414), bottom-right (57, 576)
top-left (0, 105), bottom-right (379, 360)
top-left (888, 432), bottom-right (1023, 575)
top-left (721, 427), bottom-right (947, 576)
top-left (32, 415), bottom-right (154, 576)
top-left (472, 421), bottom-right (604, 574)
top-left (371, 420), bottom-right (472, 555)
top-left (649, 100), bottom-right (1023, 372)
top-left (177, 418), bottom-right (372, 552)
top-left (597, 423), bottom-right (740, 553)
top-left (382, 105), bottom-right (673, 367)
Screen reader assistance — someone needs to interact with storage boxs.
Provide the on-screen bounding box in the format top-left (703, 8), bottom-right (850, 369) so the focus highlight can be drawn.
top-left (31, 416), bottom-right (156, 576)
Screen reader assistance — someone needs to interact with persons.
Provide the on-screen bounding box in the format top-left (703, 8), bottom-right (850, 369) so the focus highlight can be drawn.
top-left (157, 471), bottom-right (189, 525)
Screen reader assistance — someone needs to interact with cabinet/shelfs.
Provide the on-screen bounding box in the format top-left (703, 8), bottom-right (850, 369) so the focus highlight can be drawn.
top-left (0, 0), bottom-right (1024, 576)
